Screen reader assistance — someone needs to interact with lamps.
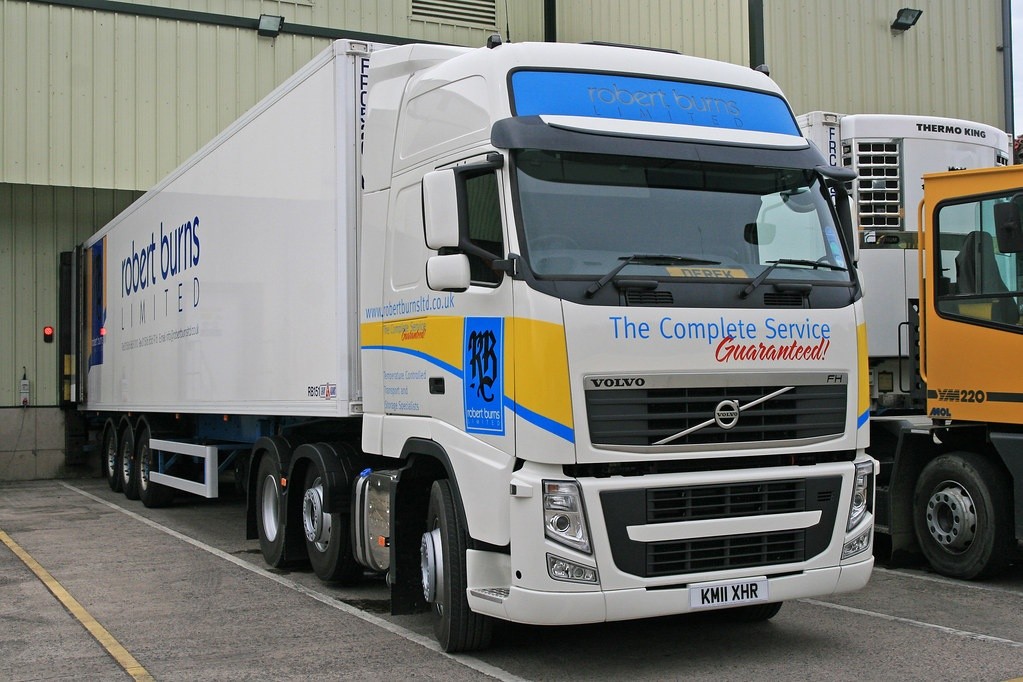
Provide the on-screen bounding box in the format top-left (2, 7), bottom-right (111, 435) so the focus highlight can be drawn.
top-left (890, 7), bottom-right (923, 30)
top-left (44, 326), bottom-right (54, 343)
top-left (257, 14), bottom-right (285, 38)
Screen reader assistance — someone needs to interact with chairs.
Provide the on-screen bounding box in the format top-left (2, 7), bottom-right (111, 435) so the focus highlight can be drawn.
top-left (955, 231), bottom-right (1019, 325)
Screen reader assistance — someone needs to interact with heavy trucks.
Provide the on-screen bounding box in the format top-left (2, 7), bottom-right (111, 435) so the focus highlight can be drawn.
top-left (71, 35), bottom-right (881, 658)
top-left (793, 110), bottom-right (1023, 585)
